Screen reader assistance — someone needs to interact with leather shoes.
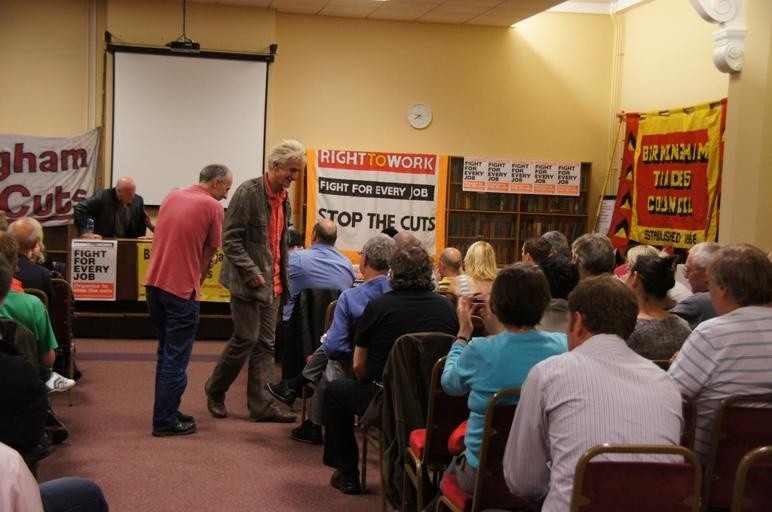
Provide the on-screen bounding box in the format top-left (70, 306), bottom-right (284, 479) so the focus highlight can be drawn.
top-left (331, 466), bottom-right (361, 495)
top-left (152, 419), bottom-right (195, 438)
top-left (204, 378), bottom-right (228, 418)
top-left (179, 410), bottom-right (195, 422)
top-left (255, 409), bottom-right (299, 423)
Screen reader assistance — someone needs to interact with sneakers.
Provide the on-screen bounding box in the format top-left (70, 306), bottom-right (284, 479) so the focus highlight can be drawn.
top-left (291, 420), bottom-right (324, 444)
top-left (45, 407), bottom-right (68, 444)
top-left (266, 379), bottom-right (298, 406)
top-left (41, 369), bottom-right (78, 392)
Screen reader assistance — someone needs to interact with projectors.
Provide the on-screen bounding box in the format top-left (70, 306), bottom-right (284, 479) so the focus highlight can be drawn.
top-left (167, 41), bottom-right (201, 53)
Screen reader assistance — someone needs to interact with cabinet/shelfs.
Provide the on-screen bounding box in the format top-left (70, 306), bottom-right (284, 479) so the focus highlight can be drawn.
top-left (445, 155), bottom-right (593, 268)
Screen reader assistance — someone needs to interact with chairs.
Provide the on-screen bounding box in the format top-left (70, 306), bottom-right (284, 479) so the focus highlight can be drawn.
top-left (275, 278), bottom-right (771, 512)
top-left (0, 267), bottom-right (75, 478)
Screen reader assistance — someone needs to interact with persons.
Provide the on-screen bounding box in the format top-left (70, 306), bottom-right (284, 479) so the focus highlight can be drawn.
top-left (142, 163), bottom-right (233, 436)
top-left (0, 209), bottom-right (110, 512)
top-left (266, 218), bottom-right (772, 512)
top-left (203, 140), bottom-right (314, 420)
top-left (73, 176), bottom-right (157, 240)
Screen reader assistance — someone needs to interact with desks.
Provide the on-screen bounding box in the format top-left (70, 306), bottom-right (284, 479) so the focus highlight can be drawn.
top-left (66, 238), bottom-right (232, 303)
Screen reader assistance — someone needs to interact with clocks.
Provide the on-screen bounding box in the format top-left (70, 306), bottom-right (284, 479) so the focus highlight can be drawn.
top-left (406, 103), bottom-right (432, 129)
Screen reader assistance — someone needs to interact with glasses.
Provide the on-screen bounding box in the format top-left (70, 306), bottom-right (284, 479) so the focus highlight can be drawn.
top-left (683, 265), bottom-right (699, 275)
top-left (357, 249), bottom-right (364, 256)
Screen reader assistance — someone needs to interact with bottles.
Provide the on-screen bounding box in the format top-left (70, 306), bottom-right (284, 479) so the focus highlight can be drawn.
top-left (86, 218), bottom-right (97, 235)
